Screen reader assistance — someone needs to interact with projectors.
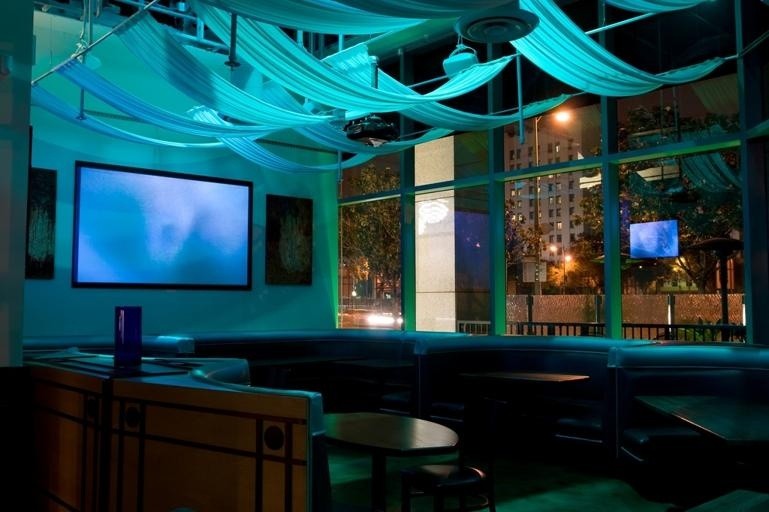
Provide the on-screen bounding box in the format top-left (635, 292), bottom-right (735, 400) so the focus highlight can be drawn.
top-left (346, 122), bottom-right (399, 147)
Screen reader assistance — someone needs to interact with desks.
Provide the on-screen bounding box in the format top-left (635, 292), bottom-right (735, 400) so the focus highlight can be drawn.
top-left (638, 393), bottom-right (768, 443)
top-left (319, 413), bottom-right (458, 511)
top-left (461, 371), bottom-right (591, 383)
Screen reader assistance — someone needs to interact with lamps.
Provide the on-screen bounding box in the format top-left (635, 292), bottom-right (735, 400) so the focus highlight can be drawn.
top-left (440, 0), bottom-right (539, 84)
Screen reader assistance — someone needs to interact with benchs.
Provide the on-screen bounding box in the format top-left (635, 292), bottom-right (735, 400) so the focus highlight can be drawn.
top-left (620, 423), bottom-right (705, 463)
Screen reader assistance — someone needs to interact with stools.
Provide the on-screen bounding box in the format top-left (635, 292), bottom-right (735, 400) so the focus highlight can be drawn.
top-left (397, 463), bottom-right (496, 510)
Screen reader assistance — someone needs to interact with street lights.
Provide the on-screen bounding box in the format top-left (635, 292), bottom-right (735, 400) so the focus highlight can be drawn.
top-left (533, 105), bottom-right (577, 258)
top-left (548, 242), bottom-right (573, 295)
